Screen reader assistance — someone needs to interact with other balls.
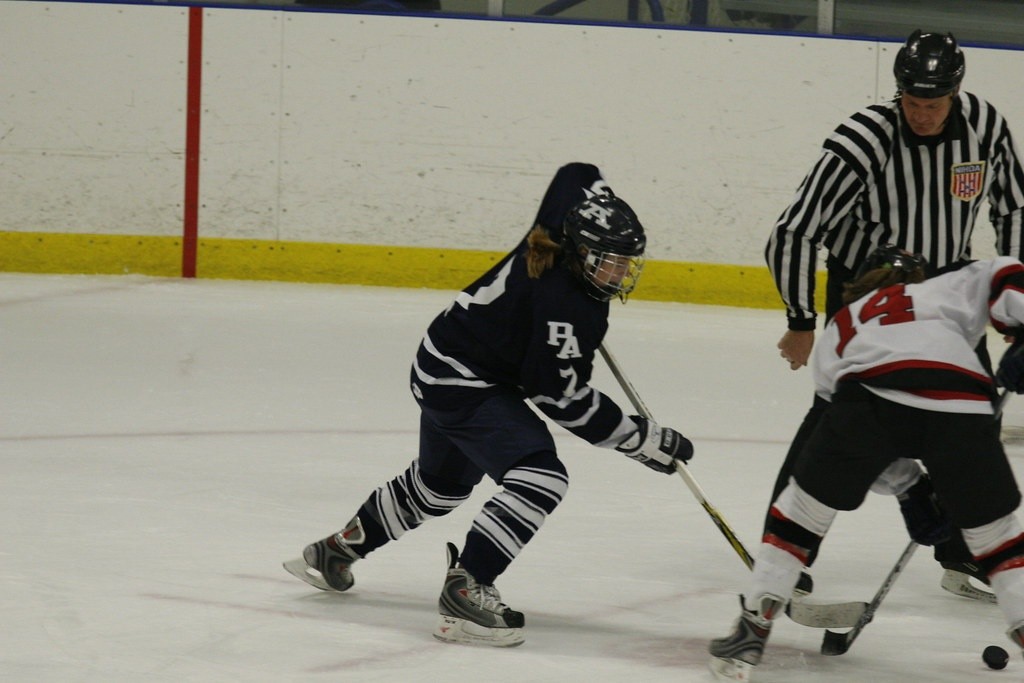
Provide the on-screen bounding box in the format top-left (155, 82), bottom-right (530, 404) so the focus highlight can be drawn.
top-left (981, 644), bottom-right (1011, 671)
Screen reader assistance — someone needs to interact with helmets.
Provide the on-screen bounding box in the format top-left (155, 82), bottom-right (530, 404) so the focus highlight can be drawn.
top-left (892, 28), bottom-right (965, 98)
top-left (853, 243), bottom-right (930, 279)
top-left (565, 195), bottom-right (646, 300)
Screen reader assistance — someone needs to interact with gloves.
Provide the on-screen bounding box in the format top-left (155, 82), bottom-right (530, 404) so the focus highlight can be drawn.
top-left (993, 332), bottom-right (1023, 394)
top-left (615, 415), bottom-right (693, 476)
top-left (896, 474), bottom-right (952, 545)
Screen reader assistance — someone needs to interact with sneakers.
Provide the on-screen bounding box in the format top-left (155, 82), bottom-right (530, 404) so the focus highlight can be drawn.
top-left (283, 516), bottom-right (366, 592)
top-left (433, 541), bottom-right (525, 647)
top-left (941, 549), bottom-right (995, 603)
top-left (708, 594), bottom-right (786, 683)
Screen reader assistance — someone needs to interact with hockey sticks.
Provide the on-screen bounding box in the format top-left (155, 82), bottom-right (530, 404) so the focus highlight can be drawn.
top-left (594, 338), bottom-right (877, 631)
top-left (817, 385), bottom-right (1016, 657)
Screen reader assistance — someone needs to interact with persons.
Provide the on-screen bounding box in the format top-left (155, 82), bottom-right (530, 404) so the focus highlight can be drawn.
top-left (282, 163), bottom-right (694, 649)
top-left (764, 27), bottom-right (1024, 603)
top-left (708, 243), bottom-right (1024, 683)
